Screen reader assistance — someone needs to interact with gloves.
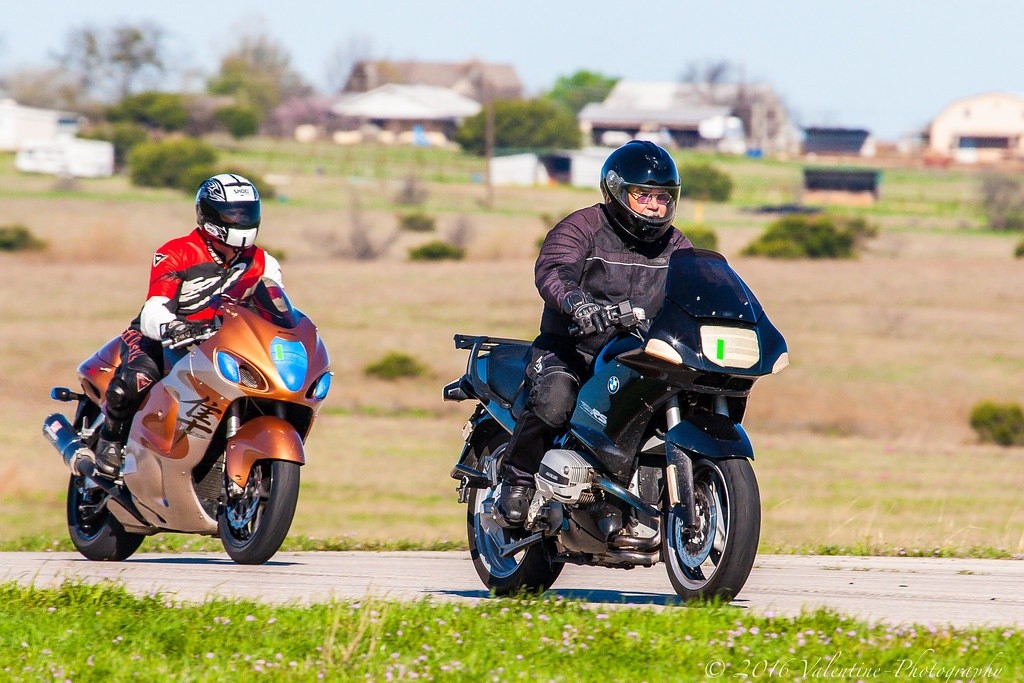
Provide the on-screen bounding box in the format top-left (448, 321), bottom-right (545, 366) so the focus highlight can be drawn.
top-left (562, 289), bottom-right (611, 336)
top-left (159, 318), bottom-right (201, 351)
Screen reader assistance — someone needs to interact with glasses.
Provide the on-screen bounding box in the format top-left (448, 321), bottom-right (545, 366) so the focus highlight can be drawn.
top-left (627, 188), bottom-right (675, 205)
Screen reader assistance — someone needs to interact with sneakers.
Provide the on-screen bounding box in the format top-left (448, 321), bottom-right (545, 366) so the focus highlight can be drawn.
top-left (502, 477), bottom-right (537, 524)
top-left (96, 429), bottom-right (122, 476)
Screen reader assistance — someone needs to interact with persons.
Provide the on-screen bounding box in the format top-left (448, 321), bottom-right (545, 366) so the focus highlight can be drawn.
top-left (96, 173), bottom-right (286, 471)
top-left (497, 139), bottom-right (695, 523)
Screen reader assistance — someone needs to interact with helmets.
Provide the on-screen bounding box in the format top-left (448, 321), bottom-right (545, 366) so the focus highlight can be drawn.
top-left (601, 140), bottom-right (683, 246)
top-left (195, 173), bottom-right (261, 249)
